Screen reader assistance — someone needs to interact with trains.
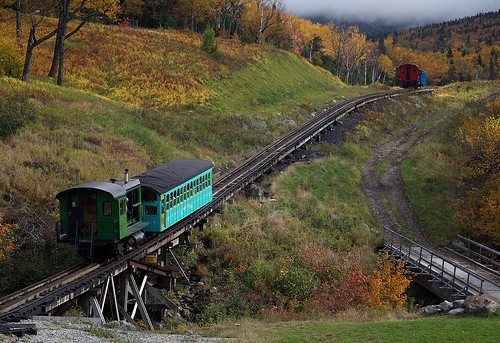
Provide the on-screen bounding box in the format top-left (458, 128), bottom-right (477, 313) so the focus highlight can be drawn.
top-left (55, 156), bottom-right (214, 259)
top-left (396, 61), bottom-right (427, 87)
